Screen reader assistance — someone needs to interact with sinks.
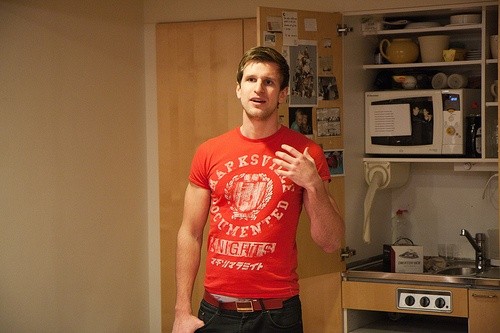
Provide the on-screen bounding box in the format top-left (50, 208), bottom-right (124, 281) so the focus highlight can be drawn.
top-left (431, 256), bottom-right (482, 278)
top-left (476, 267), bottom-right (500, 279)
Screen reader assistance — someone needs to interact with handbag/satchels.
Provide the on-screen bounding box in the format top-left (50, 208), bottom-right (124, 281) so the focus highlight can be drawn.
top-left (383, 238), bottom-right (423, 274)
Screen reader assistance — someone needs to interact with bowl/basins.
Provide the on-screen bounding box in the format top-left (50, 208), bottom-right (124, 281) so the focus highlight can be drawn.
top-left (450, 14), bottom-right (481, 25)
top-left (404, 21), bottom-right (442, 28)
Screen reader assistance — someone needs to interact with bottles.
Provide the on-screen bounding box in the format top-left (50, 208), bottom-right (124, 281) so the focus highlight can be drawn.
top-left (465, 113), bottom-right (481, 158)
top-left (374, 42), bottom-right (386, 64)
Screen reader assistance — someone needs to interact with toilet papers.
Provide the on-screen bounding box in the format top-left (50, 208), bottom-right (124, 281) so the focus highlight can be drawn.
top-left (362, 162), bottom-right (411, 244)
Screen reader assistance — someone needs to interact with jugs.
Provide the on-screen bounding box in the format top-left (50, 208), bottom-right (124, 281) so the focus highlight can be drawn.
top-left (486, 229), bottom-right (500, 266)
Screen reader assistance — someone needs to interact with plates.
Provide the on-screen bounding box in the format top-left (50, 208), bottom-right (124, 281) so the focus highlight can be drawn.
top-left (462, 49), bottom-right (481, 60)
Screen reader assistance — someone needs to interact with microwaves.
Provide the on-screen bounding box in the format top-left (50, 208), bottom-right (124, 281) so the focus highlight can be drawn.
top-left (364, 88), bottom-right (481, 155)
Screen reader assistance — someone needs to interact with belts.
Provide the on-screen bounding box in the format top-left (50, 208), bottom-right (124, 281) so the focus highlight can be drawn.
top-left (203, 290), bottom-right (290, 313)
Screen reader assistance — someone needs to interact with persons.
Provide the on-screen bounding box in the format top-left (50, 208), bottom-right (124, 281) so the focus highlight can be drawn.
top-left (412, 106), bottom-right (432, 144)
top-left (292, 111), bottom-right (313, 135)
top-left (172, 47), bottom-right (345, 333)
top-left (318, 77), bottom-right (338, 101)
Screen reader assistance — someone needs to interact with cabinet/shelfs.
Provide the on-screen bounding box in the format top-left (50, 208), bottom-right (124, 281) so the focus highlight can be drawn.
top-left (257, 0), bottom-right (500, 178)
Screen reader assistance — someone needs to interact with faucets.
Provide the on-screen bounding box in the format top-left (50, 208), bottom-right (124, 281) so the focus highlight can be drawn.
top-left (460, 228), bottom-right (486, 271)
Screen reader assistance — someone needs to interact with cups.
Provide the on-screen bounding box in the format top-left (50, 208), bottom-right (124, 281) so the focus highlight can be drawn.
top-left (491, 79), bottom-right (498, 102)
top-left (417, 36), bottom-right (450, 63)
top-left (438, 244), bottom-right (454, 264)
top-left (490, 35), bottom-right (497, 59)
top-left (378, 38), bottom-right (419, 63)
